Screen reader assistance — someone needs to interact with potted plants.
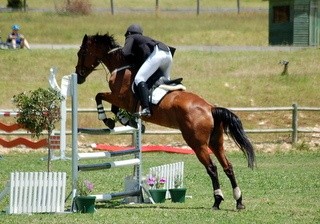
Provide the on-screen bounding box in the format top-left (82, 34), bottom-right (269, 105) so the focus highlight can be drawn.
top-left (168, 173), bottom-right (196, 203)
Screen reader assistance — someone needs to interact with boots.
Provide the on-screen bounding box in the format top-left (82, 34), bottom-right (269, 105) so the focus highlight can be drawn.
top-left (133, 81), bottom-right (151, 116)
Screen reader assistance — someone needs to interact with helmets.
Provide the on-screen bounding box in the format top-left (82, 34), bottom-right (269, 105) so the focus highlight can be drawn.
top-left (125, 24), bottom-right (143, 37)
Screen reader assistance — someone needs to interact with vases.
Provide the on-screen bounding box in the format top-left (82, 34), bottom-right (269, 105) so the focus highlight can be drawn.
top-left (148, 190), bottom-right (167, 203)
top-left (75, 196), bottom-right (96, 214)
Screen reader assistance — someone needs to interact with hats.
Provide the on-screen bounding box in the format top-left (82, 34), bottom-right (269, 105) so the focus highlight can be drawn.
top-left (12, 24), bottom-right (20, 29)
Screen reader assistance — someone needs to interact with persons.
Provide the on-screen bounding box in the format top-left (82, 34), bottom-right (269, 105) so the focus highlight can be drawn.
top-left (119, 25), bottom-right (176, 119)
top-left (6, 25), bottom-right (33, 50)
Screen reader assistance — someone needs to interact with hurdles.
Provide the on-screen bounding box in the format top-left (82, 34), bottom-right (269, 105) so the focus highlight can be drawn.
top-left (70, 73), bottom-right (143, 210)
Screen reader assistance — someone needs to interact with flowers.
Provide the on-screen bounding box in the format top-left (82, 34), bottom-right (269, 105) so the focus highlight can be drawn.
top-left (78, 179), bottom-right (95, 197)
top-left (146, 173), bottom-right (166, 190)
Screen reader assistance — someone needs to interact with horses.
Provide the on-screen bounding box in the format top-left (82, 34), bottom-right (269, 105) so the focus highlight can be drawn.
top-left (75, 31), bottom-right (256, 210)
